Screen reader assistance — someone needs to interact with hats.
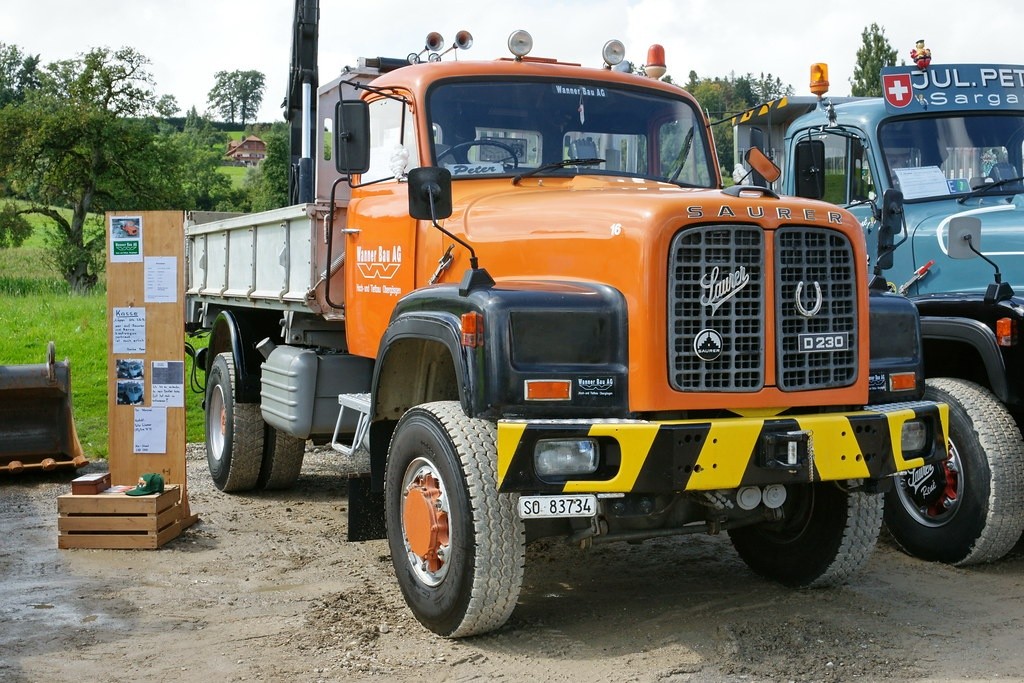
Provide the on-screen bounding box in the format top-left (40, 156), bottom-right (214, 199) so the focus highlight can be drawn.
top-left (124, 473), bottom-right (165, 496)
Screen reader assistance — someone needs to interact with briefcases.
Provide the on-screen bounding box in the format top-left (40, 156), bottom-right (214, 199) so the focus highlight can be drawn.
top-left (71, 473), bottom-right (112, 494)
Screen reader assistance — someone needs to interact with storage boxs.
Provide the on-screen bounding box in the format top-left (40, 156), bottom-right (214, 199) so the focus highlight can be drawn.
top-left (71, 473), bottom-right (112, 495)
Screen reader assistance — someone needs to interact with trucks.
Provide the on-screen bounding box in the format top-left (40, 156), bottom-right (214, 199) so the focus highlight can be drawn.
top-left (182, 30), bottom-right (954, 639)
top-left (729, 37), bottom-right (1023, 568)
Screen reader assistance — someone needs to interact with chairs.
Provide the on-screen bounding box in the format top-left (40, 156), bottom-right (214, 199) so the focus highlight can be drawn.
top-left (989, 163), bottom-right (1019, 190)
